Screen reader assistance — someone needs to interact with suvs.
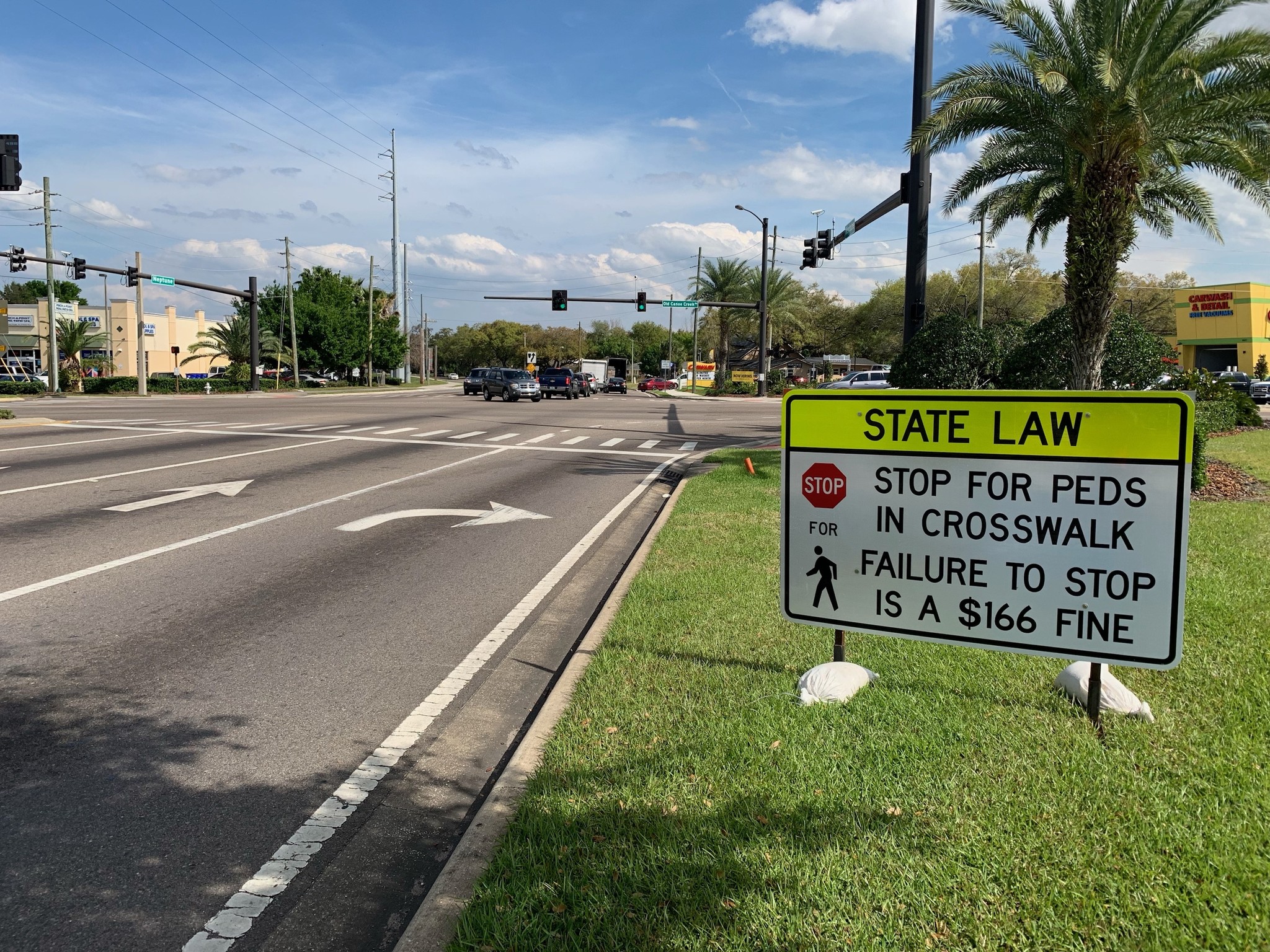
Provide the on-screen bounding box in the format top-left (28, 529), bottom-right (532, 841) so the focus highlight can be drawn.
top-left (463, 367), bottom-right (542, 403)
top-left (817, 369), bottom-right (901, 390)
top-left (1137, 371), bottom-right (1270, 404)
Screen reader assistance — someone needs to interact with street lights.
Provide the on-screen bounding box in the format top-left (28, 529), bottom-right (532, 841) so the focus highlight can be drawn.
top-left (735, 204), bottom-right (769, 397)
top-left (957, 294), bottom-right (968, 319)
top-left (697, 346), bottom-right (702, 362)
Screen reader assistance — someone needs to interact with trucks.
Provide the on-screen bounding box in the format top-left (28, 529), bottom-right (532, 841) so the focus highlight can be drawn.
top-left (581, 355), bottom-right (628, 392)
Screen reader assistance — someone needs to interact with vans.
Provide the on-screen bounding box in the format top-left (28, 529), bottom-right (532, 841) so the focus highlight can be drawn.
top-left (207, 367), bottom-right (227, 378)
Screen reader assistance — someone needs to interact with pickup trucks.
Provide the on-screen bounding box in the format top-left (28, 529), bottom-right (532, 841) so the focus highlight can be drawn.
top-left (536, 367), bottom-right (581, 400)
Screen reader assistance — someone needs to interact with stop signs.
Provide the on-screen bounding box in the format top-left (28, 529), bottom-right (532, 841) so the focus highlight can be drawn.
top-left (802, 462), bottom-right (846, 509)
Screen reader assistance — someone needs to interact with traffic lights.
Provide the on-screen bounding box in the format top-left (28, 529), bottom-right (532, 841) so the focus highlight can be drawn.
top-left (74, 258), bottom-right (87, 279)
top-left (637, 292), bottom-right (646, 312)
top-left (10, 244), bottom-right (28, 272)
top-left (551, 290), bottom-right (568, 312)
top-left (171, 347), bottom-right (179, 354)
top-left (803, 238), bottom-right (816, 267)
top-left (817, 229), bottom-right (831, 260)
top-left (128, 266), bottom-right (139, 287)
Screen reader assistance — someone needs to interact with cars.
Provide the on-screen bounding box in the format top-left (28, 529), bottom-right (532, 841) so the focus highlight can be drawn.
top-left (448, 373), bottom-right (459, 380)
top-left (785, 375), bottom-right (808, 386)
top-left (573, 371), bottom-right (599, 397)
top-left (258, 367), bottom-right (360, 388)
top-left (637, 378), bottom-right (675, 392)
top-left (0, 365), bottom-right (60, 390)
top-left (603, 378), bottom-right (627, 394)
top-left (667, 373), bottom-right (688, 389)
